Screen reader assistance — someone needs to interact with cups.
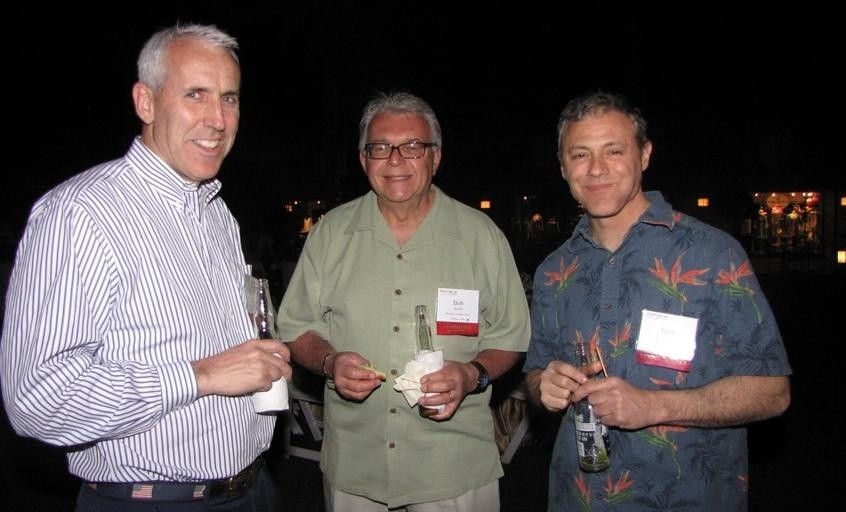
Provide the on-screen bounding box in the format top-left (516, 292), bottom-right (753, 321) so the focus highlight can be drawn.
top-left (742, 214), bottom-right (811, 247)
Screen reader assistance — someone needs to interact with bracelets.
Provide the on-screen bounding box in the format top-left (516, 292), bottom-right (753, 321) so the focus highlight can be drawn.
top-left (319, 349), bottom-right (332, 376)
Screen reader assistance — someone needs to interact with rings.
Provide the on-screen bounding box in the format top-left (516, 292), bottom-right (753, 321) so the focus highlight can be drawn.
top-left (448, 391), bottom-right (452, 402)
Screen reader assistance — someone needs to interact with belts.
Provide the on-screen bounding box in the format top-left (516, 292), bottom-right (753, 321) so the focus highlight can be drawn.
top-left (80, 448), bottom-right (272, 500)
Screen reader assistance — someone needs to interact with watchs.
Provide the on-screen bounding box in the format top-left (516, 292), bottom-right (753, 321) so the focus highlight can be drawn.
top-left (470, 362), bottom-right (490, 394)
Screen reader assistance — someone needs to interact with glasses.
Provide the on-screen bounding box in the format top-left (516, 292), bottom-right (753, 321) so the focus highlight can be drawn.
top-left (361, 139), bottom-right (437, 160)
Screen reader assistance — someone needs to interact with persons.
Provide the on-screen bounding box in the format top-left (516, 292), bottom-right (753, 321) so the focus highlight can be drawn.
top-left (276, 88), bottom-right (533, 512)
top-left (1, 20), bottom-right (293, 509)
top-left (527, 88), bottom-right (792, 511)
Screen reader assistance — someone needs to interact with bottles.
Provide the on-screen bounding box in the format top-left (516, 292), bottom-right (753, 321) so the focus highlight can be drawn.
top-left (250, 278), bottom-right (289, 411)
top-left (569, 343), bottom-right (613, 472)
top-left (415, 306), bottom-right (445, 418)
